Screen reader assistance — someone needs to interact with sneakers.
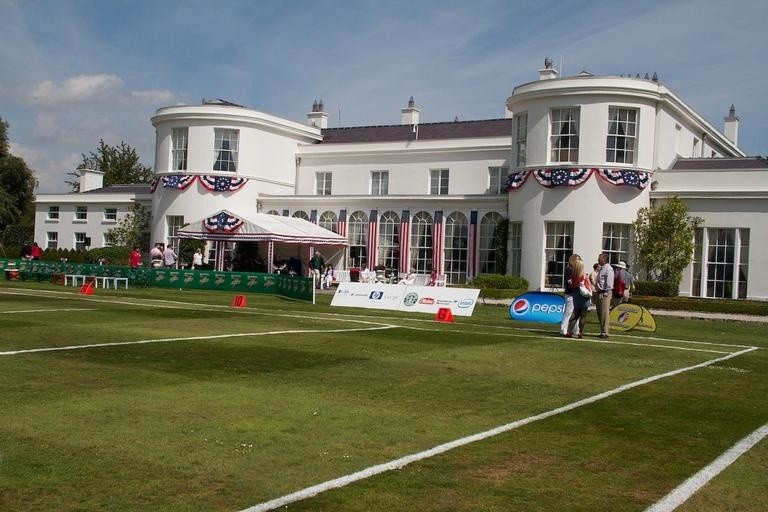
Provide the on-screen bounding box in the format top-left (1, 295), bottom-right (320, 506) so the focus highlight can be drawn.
top-left (561, 331), bottom-right (582, 338)
top-left (599, 335), bottom-right (608, 339)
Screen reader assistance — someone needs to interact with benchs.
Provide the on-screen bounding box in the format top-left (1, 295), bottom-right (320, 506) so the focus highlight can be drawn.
top-left (320, 269), bottom-right (377, 290)
top-left (399, 273), bottom-right (447, 288)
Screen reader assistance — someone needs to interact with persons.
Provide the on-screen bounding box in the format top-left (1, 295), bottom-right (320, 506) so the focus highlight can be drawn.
top-left (149, 243), bottom-right (164, 269)
top-left (128, 245), bottom-right (143, 271)
top-left (188, 247), bottom-right (203, 272)
top-left (162, 245), bottom-right (178, 269)
top-left (559, 254), bottom-right (583, 338)
top-left (21, 242), bottom-right (31, 283)
top-left (594, 253), bottom-right (613, 339)
top-left (562, 259), bottom-right (589, 338)
top-left (308, 252), bottom-right (337, 289)
top-left (589, 261), bottom-right (635, 310)
top-left (30, 242), bottom-right (44, 284)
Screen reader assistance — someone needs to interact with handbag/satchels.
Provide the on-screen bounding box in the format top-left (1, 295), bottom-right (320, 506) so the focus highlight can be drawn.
top-left (579, 282), bottom-right (592, 298)
top-left (613, 280), bottom-right (625, 298)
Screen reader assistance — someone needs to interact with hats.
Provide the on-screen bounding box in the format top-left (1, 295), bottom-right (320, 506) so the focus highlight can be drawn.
top-left (616, 262), bottom-right (626, 268)
